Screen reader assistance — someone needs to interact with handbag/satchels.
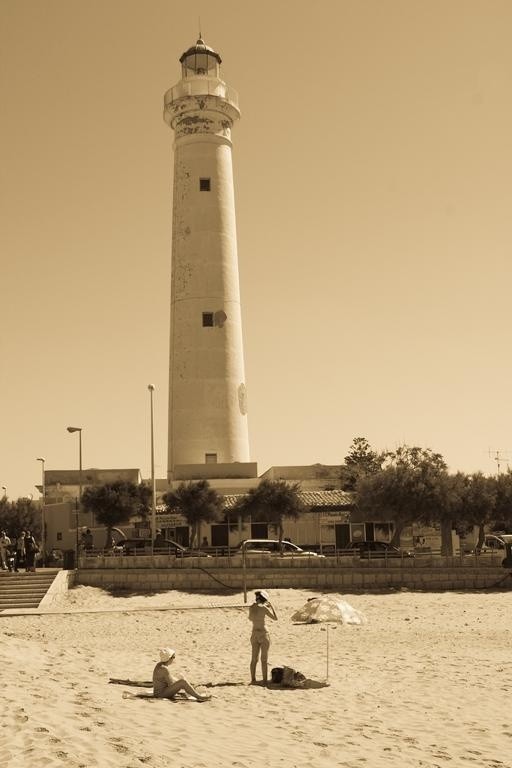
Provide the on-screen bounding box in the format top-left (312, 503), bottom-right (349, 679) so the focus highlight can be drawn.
top-left (29, 543), bottom-right (40, 553)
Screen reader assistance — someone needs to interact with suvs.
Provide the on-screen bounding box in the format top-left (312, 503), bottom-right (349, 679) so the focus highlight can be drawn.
top-left (236, 539), bottom-right (325, 558)
top-left (343, 541), bottom-right (416, 558)
top-left (115, 537), bottom-right (211, 558)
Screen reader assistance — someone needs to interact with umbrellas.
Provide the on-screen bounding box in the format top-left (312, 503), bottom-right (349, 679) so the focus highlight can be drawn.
top-left (290, 594), bottom-right (369, 680)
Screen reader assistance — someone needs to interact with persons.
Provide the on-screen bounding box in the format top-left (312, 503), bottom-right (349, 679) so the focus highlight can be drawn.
top-left (84, 529), bottom-right (95, 556)
top-left (1, 529), bottom-right (13, 570)
top-left (153, 647), bottom-right (213, 702)
top-left (154, 528), bottom-right (164, 548)
top-left (14, 530), bottom-right (28, 572)
top-left (247, 589), bottom-right (279, 685)
top-left (78, 532), bottom-right (87, 551)
top-left (414, 539), bottom-right (422, 548)
top-left (420, 538), bottom-right (428, 547)
top-left (24, 531), bottom-right (37, 572)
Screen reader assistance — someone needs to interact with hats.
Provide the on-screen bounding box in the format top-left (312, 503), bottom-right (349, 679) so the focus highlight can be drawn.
top-left (159, 647), bottom-right (174, 663)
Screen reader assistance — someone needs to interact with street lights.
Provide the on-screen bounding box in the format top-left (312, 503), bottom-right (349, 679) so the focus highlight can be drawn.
top-left (35, 458), bottom-right (46, 566)
top-left (2, 486), bottom-right (7, 497)
top-left (67, 425), bottom-right (84, 501)
top-left (147, 384), bottom-right (158, 556)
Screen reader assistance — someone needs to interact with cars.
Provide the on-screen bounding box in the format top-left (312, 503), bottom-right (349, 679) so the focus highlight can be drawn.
top-left (8, 547), bottom-right (45, 568)
